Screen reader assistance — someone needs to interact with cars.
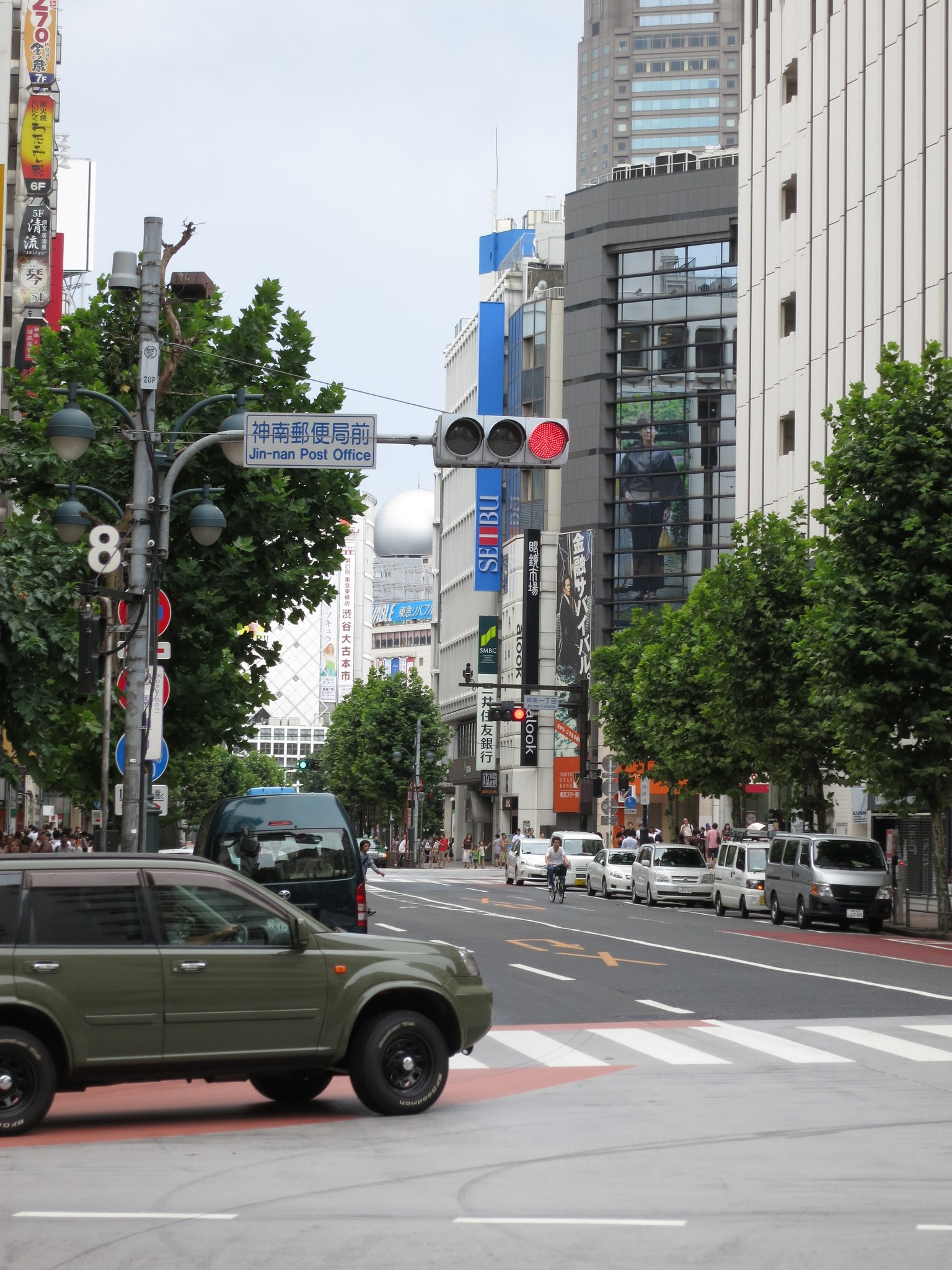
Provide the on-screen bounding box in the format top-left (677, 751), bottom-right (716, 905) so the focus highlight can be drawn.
top-left (504, 838), bottom-right (551, 885)
top-left (630, 844), bottom-right (714, 907)
top-left (586, 848), bottom-right (639, 899)
top-left (356, 835), bottom-right (387, 868)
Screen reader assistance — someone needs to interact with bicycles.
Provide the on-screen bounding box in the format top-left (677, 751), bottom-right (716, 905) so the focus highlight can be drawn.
top-left (546, 864), bottom-right (570, 904)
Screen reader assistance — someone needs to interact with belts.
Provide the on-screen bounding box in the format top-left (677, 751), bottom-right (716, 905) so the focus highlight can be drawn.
top-left (684, 836), bottom-right (691, 837)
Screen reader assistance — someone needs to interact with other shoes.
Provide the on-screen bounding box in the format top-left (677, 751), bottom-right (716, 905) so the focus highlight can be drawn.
top-left (495, 865), bottom-right (498, 867)
top-left (474, 866), bottom-right (477, 868)
top-left (548, 885), bottom-right (553, 889)
top-left (439, 866), bottom-right (445, 869)
top-left (500, 867), bottom-right (502, 869)
top-left (467, 866), bottom-right (470, 868)
top-left (481, 866), bottom-right (485, 869)
top-left (434, 866), bottom-right (440, 868)
top-left (560, 893), bottom-right (566, 898)
top-left (367, 907), bottom-right (376, 916)
top-left (505, 867), bottom-right (506, 869)
top-left (464, 865), bottom-right (467, 868)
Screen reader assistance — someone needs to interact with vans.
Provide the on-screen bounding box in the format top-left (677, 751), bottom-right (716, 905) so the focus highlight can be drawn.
top-left (764, 833), bottom-right (898, 934)
top-left (549, 831), bottom-right (606, 891)
top-left (713, 828), bottom-right (789, 919)
top-left (193, 784), bottom-right (369, 936)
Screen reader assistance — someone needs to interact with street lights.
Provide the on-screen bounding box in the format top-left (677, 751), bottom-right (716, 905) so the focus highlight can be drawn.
top-left (25, 383), bottom-right (268, 856)
top-left (393, 746), bottom-right (437, 866)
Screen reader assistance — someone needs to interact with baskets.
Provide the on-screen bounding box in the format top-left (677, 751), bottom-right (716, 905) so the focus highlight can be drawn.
top-left (554, 867), bottom-right (567, 876)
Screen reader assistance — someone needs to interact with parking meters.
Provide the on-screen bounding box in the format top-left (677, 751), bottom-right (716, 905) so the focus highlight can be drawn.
top-left (897, 860), bottom-right (905, 925)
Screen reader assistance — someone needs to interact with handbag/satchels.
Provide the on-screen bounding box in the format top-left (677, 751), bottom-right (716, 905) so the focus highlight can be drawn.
top-left (617, 837), bottom-right (623, 843)
top-left (716, 830), bottom-right (721, 844)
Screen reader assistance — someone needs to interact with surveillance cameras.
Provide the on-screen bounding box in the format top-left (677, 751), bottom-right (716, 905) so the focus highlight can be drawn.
top-left (465, 673), bottom-right (471, 678)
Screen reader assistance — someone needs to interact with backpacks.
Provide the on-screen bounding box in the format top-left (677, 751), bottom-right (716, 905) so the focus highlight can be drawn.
top-left (424, 840), bottom-right (430, 851)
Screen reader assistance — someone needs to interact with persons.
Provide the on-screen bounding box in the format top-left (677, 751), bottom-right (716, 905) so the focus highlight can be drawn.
top-left (610, 822), bottom-right (642, 850)
top-left (417, 833), bottom-right (454, 869)
top-left (158, 887), bottom-right (239, 945)
top-left (677, 818), bottom-right (732, 869)
top-left (545, 837), bottom-right (570, 897)
top-left (392, 835), bottom-right (409, 868)
top-left (647, 827), bottom-right (663, 844)
top-left (525, 828), bottom-right (536, 838)
top-left (539, 832), bottom-right (545, 839)
top-left (462, 834), bottom-right (491, 868)
top-left (360, 840), bottom-right (385, 916)
top-left (0, 825), bottom-right (93, 854)
top-left (493, 834), bottom-right (501, 867)
top-left (744, 852), bottom-right (757, 869)
top-left (596, 832), bottom-right (605, 848)
top-left (512, 828), bottom-right (525, 844)
top-left (499, 833), bottom-right (510, 869)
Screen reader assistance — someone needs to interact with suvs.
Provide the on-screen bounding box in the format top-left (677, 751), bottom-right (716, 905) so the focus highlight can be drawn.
top-left (0, 852), bottom-right (494, 1136)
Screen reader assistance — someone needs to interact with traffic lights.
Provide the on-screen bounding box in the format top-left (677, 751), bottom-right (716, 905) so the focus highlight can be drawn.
top-left (436, 412), bottom-right (570, 474)
top-left (489, 707), bottom-right (525, 722)
top-left (298, 761), bottom-right (319, 769)
top-left (76, 613), bottom-right (105, 695)
top-left (428, 792), bottom-right (434, 806)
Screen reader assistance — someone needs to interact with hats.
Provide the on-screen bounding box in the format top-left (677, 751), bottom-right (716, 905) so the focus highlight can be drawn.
top-left (38, 830), bottom-right (46, 835)
top-left (46, 832), bottom-right (51, 837)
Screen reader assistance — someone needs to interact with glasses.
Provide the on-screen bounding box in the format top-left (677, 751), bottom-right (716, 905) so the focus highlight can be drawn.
top-left (706, 827), bottom-right (710, 829)
